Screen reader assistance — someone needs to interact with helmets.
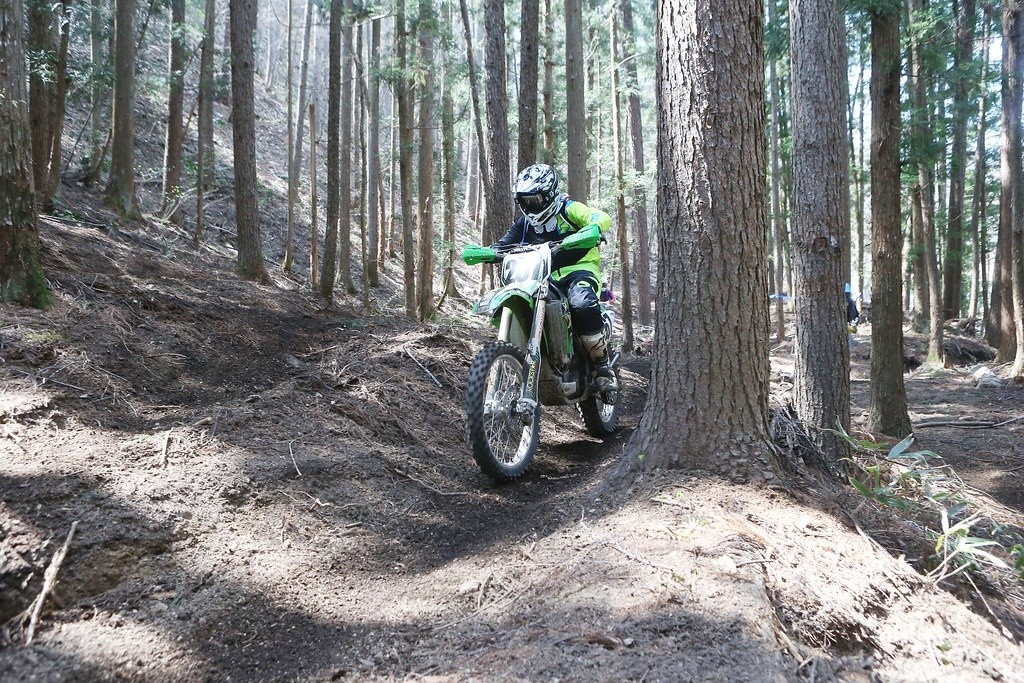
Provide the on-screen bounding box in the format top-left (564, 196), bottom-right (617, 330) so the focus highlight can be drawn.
top-left (601, 277), bottom-right (608, 291)
top-left (514, 164), bottom-right (564, 226)
top-left (845, 283), bottom-right (851, 292)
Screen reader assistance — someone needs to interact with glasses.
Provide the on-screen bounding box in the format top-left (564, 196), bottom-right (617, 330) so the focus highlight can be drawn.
top-left (602, 283), bottom-right (607, 288)
top-left (522, 194), bottom-right (543, 204)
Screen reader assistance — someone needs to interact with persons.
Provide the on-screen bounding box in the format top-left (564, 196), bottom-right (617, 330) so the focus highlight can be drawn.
top-left (479, 162), bottom-right (619, 395)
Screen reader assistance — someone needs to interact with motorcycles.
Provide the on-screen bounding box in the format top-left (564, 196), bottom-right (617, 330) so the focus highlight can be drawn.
top-left (462, 223), bottom-right (622, 485)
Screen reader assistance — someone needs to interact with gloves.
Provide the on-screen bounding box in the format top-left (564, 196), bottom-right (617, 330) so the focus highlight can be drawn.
top-left (611, 300), bottom-right (614, 303)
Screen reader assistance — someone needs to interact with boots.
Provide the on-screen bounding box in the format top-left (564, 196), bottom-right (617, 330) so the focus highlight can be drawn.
top-left (580, 332), bottom-right (619, 391)
